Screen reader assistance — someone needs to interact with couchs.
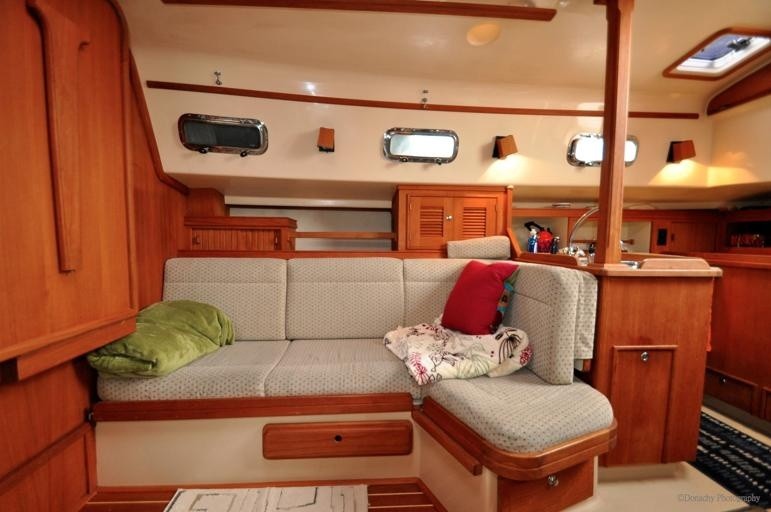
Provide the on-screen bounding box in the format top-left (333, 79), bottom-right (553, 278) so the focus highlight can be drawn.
top-left (88, 250), bottom-right (618, 512)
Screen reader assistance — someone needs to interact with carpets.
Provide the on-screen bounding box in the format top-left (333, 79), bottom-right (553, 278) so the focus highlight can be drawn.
top-left (684, 410), bottom-right (771, 512)
top-left (162, 485), bottom-right (368, 512)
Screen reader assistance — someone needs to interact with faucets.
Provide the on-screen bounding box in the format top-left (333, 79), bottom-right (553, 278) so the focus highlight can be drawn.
top-left (568, 205), bottom-right (600, 255)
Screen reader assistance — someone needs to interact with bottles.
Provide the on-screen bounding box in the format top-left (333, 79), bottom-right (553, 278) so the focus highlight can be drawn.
top-left (549, 238), bottom-right (559, 253)
top-left (528, 229), bottom-right (538, 253)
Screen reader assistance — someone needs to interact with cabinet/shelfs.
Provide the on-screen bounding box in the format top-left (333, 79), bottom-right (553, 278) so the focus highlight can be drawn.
top-left (184, 216), bottom-right (297, 250)
top-left (392, 184), bottom-right (513, 250)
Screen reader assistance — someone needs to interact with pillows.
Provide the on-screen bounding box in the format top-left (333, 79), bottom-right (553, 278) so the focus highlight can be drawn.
top-left (441, 259), bottom-right (521, 336)
